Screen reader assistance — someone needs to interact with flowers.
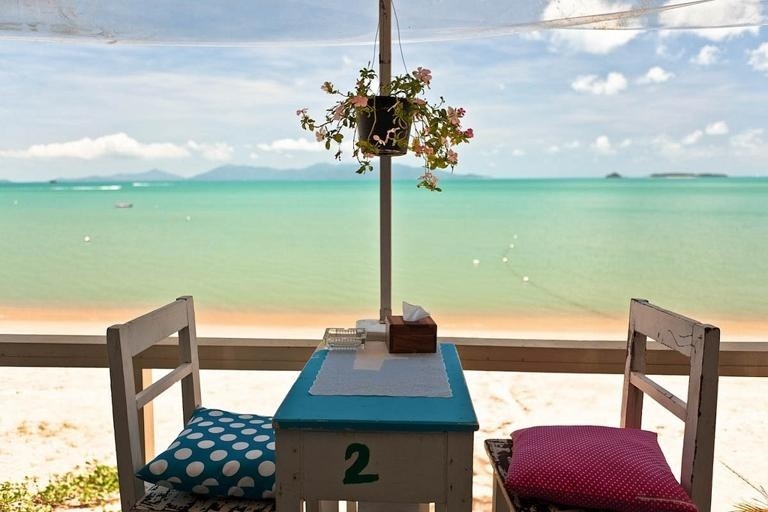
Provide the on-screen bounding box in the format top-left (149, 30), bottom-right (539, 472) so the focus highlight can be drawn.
top-left (296, 61), bottom-right (474, 192)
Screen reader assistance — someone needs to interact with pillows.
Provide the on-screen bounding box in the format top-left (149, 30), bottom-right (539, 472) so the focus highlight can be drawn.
top-left (135, 404), bottom-right (276, 501)
top-left (504, 425), bottom-right (700, 512)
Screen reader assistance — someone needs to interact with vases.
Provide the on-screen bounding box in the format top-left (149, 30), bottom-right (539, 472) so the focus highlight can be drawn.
top-left (355, 95), bottom-right (413, 156)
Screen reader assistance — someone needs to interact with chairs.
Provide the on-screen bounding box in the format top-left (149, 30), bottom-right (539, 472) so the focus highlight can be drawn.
top-left (106, 296), bottom-right (276, 512)
top-left (483, 298), bottom-right (720, 512)
